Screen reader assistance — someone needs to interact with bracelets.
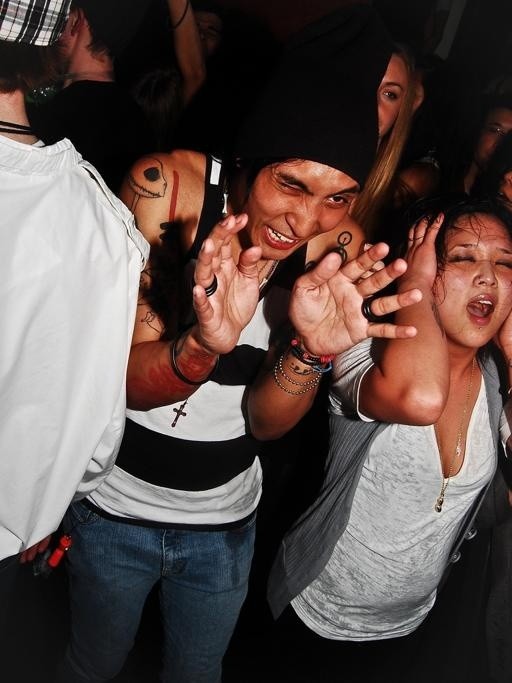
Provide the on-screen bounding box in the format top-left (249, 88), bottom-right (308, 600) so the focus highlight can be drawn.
top-left (170, 326), bottom-right (223, 386)
top-left (273, 349), bottom-right (321, 395)
top-left (291, 338), bottom-right (335, 373)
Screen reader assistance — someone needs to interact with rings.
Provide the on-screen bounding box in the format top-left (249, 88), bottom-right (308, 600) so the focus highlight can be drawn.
top-left (361, 295), bottom-right (382, 321)
top-left (203, 272), bottom-right (218, 296)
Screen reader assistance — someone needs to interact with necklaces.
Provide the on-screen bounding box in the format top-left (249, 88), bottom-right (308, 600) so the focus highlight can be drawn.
top-left (435, 349), bottom-right (478, 515)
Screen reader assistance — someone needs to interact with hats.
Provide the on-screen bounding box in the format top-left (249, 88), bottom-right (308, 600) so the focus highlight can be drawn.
top-left (251, 19), bottom-right (391, 190)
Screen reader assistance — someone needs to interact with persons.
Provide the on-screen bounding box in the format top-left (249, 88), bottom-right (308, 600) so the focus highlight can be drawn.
top-left (242, 192), bottom-right (511, 679)
top-left (2, 2), bottom-right (512, 575)
top-left (63, 48), bottom-right (429, 678)
top-left (0, 31), bottom-right (154, 682)
